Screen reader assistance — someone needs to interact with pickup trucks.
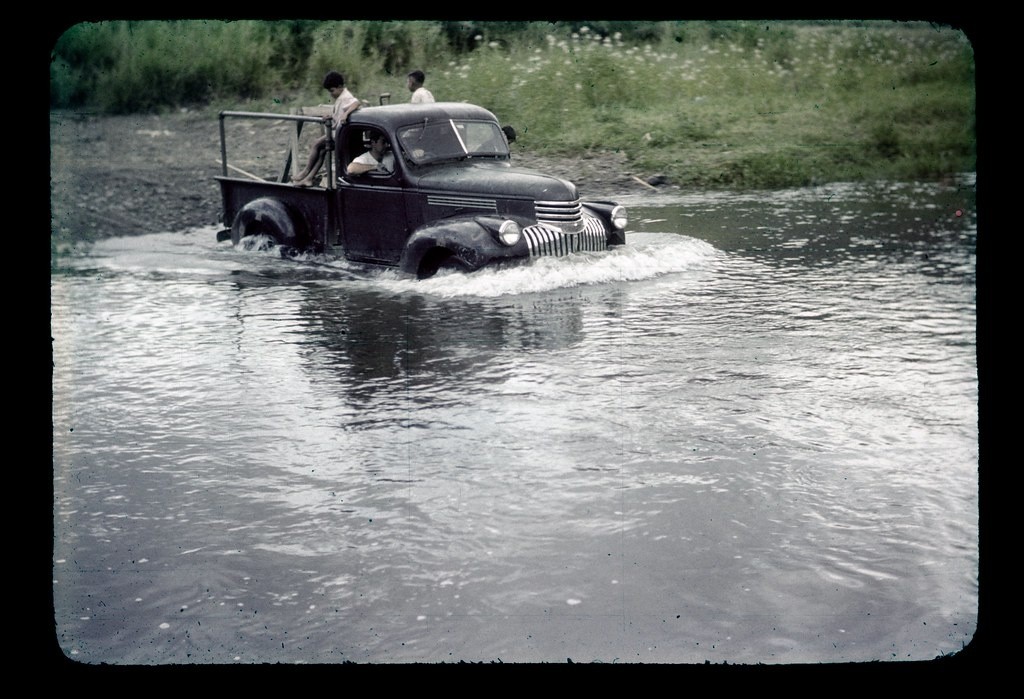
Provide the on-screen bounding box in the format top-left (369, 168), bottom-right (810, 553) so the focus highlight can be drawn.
top-left (211, 94), bottom-right (628, 280)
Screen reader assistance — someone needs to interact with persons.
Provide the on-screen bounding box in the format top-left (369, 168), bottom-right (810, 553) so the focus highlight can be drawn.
top-left (291, 73), bottom-right (360, 187)
top-left (345, 129), bottom-right (425, 175)
top-left (402, 71), bottom-right (434, 137)
